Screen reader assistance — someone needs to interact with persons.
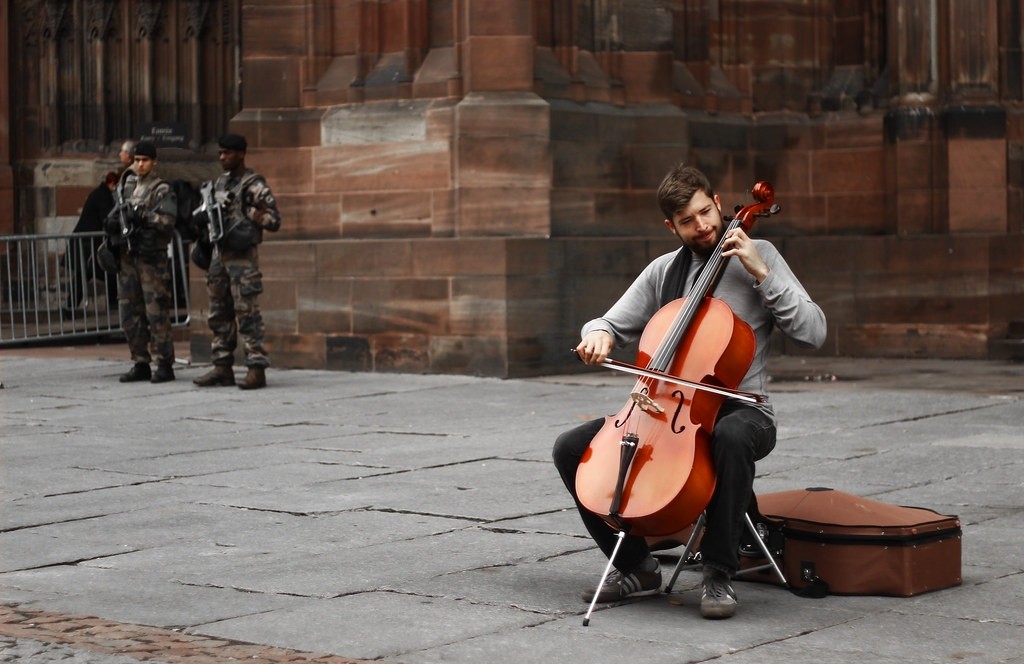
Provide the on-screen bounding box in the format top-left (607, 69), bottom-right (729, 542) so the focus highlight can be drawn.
top-left (192, 133), bottom-right (281, 390)
top-left (104, 141), bottom-right (177, 383)
top-left (60, 140), bottom-right (201, 323)
top-left (551, 163), bottom-right (828, 618)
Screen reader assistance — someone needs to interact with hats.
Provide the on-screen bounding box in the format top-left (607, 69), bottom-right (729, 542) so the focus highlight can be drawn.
top-left (219, 134), bottom-right (250, 151)
top-left (132, 142), bottom-right (156, 159)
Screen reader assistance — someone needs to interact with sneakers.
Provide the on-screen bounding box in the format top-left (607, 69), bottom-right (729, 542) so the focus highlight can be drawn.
top-left (118, 363), bottom-right (151, 382)
top-left (236, 365), bottom-right (266, 389)
top-left (580, 558), bottom-right (661, 601)
top-left (700, 565), bottom-right (737, 617)
top-left (150, 369), bottom-right (176, 383)
top-left (192, 364), bottom-right (236, 386)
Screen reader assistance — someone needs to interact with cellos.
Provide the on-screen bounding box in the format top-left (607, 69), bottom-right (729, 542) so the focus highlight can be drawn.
top-left (574, 178), bottom-right (781, 627)
